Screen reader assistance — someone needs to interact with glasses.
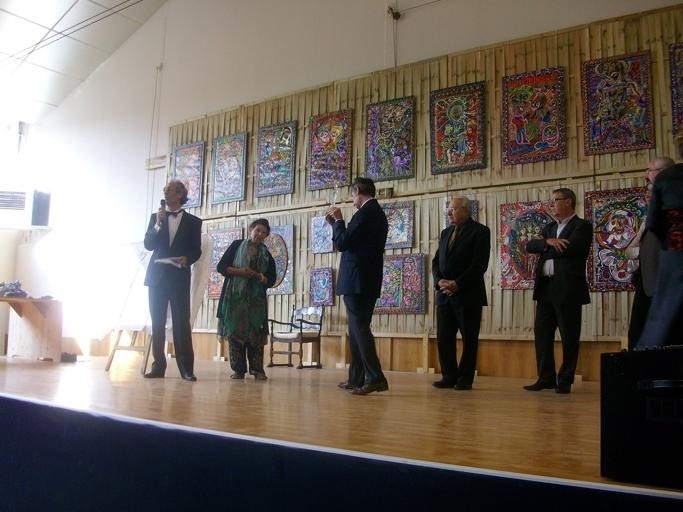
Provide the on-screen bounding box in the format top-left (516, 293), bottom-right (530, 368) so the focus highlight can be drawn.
top-left (553, 197), bottom-right (569, 203)
top-left (645, 166), bottom-right (665, 173)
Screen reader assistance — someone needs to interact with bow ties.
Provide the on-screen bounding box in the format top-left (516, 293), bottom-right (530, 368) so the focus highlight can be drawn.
top-left (166, 210), bottom-right (184, 216)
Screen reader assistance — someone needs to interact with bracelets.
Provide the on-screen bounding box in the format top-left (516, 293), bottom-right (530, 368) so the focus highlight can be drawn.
top-left (257, 272), bottom-right (266, 284)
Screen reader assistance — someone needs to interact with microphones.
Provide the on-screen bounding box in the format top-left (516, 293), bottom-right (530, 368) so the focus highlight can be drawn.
top-left (161, 200), bottom-right (165, 221)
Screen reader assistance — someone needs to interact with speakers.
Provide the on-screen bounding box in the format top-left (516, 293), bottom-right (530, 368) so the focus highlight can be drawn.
top-left (595, 345), bottom-right (682, 488)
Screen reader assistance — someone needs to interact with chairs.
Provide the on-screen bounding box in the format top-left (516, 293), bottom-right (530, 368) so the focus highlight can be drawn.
top-left (268, 305), bottom-right (325, 369)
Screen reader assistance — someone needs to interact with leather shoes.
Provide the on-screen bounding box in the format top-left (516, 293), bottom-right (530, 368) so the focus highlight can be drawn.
top-left (523, 378), bottom-right (557, 390)
top-left (144, 369), bottom-right (166, 377)
top-left (556, 380), bottom-right (573, 394)
top-left (252, 374), bottom-right (267, 379)
top-left (230, 371), bottom-right (245, 379)
top-left (454, 382), bottom-right (473, 390)
top-left (338, 381), bottom-right (364, 388)
top-left (182, 373), bottom-right (198, 380)
top-left (349, 381), bottom-right (388, 394)
top-left (432, 378), bottom-right (458, 388)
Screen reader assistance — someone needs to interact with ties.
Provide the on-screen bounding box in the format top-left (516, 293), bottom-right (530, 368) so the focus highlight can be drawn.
top-left (448, 226), bottom-right (458, 247)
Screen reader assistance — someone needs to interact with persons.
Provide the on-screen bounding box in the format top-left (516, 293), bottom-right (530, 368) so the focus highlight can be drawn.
top-left (324, 177), bottom-right (389, 395)
top-left (625, 182), bottom-right (654, 350)
top-left (523, 188), bottom-right (591, 394)
top-left (431, 196), bottom-right (491, 391)
top-left (636, 132), bottom-right (683, 348)
top-left (640, 157), bottom-right (676, 296)
top-left (144, 180), bottom-right (202, 382)
top-left (217, 219), bottom-right (276, 380)
